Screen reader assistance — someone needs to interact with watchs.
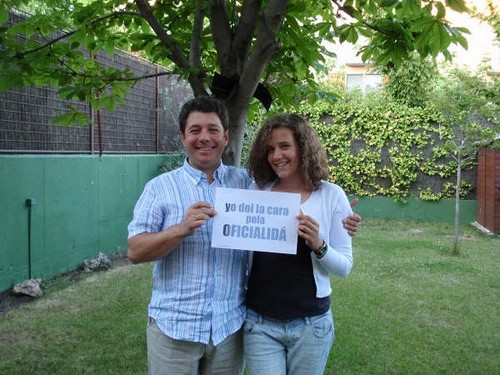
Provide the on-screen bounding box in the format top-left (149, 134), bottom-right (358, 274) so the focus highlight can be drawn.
top-left (313, 240), bottom-right (328, 256)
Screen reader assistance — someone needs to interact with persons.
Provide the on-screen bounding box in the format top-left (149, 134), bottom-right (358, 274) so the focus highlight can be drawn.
top-left (241, 111), bottom-right (355, 375)
top-left (124, 96), bottom-right (362, 375)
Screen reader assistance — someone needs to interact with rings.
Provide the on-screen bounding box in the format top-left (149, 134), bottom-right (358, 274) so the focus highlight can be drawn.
top-left (195, 214), bottom-right (198, 220)
